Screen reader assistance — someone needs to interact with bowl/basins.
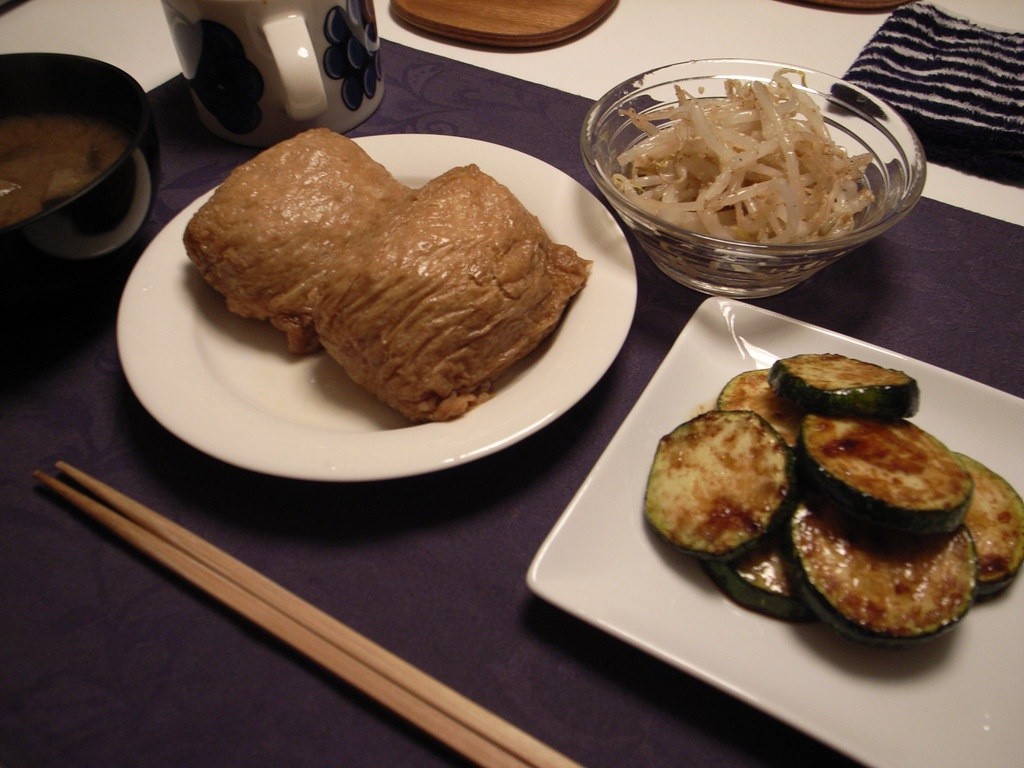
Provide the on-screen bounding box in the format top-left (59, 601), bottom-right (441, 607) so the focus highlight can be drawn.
top-left (580, 55), bottom-right (926, 299)
top-left (0, 51), bottom-right (157, 333)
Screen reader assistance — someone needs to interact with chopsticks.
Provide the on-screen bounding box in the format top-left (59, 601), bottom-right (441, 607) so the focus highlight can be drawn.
top-left (27, 458), bottom-right (579, 768)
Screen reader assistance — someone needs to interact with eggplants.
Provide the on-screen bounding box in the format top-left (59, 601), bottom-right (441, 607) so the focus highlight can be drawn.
top-left (641, 349), bottom-right (1024, 645)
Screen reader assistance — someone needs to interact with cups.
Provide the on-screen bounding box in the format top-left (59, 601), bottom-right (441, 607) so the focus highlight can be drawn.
top-left (161, 0), bottom-right (382, 142)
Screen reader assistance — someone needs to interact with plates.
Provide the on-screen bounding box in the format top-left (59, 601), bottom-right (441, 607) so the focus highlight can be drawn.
top-left (115, 135), bottom-right (639, 483)
top-left (525, 297), bottom-right (1023, 767)
